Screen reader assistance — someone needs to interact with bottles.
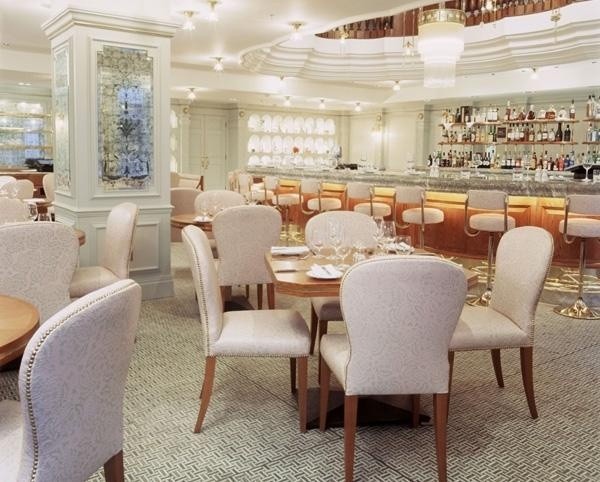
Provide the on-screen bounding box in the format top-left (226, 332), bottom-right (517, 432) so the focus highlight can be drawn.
top-left (511, 151), bottom-right (517, 168)
top-left (536, 124), bottom-right (544, 143)
top-left (537, 106), bottom-right (547, 119)
top-left (516, 153), bottom-right (520, 169)
top-left (476, 125), bottom-right (481, 143)
top-left (443, 107), bottom-right (461, 124)
top-left (480, 126), bottom-right (485, 143)
top-left (488, 127), bottom-right (493, 144)
top-left (488, 104), bottom-right (494, 122)
top-left (483, 152), bottom-right (487, 168)
top-left (510, 106), bottom-right (519, 120)
top-left (555, 123), bottom-right (562, 142)
top-left (427, 154), bottom-right (432, 167)
top-left (592, 151), bottom-right (596, 164)
top-left (524, 123), bottom-right (529, 143)
top-left (511, 124), bottom-right (517, 142)
top-left (526, 105), bottom-right (536, 121)
top-left (570, 100), bottom-right (576, 119)
top-left (528, 124), bottom-right (535, 143)
top-left (564, 155), bottom-right (570, 170)
top-left (441, 151), bottom-right (446, 168)
top-left (580, 152), bottom-right (585, 164)
top-left (592, 151), bottom-right (597, 164)
top-left (507, 123), bottom-right (513, 142)
top-left (454, 150), bottom-right (458, 168)
top-left (495, 154), bottom-right (501, 169)
top-left (470, 108), bottom-right (475, 122)
top-left (518, 106), bottom-right (526, 120)
top-left (586, 123), bottom-right (593, 142)
top-left (492, 105), bottom-right (499, 123)
top-left (462, 127), bottom-right (467, 145)
top-left (585, 95), bottom-right (592, 120)
top-left (454, 131), bottom-right (458, 143)
top-left (570, 152), bottom-right (575, 167)
top-left (481, 107), bottom-right (487, 123)
top-left (441, 126), bottom-right (446, 144)
top-left (592, 95), bottom-right (597, 120)
top-left (505, 100), bottom-right (511, 121)
top-left (558, 106), bottom-right (568, 119)
top-left (548, 128), bottom-right (556, 143)
top-left (543, 151), bottom-right (548, 169)
top-left (448, 150), bottom-right (452, 167)
top-left (519, 123), bottom-right (525, 142)
top-left (475, 108), bottom-right (481, 122)
top-left (506, 151), bottom-right (512, 168)
top-left (515, 124), bottom-right (521, 142)
top-left (542, 123), bottom-right (548, 142)
top-left (458, 151), bottom-right (466, 168)
top-left (559, 155), bottom-right (564, 171)
top-left (467, 128), bottom-right (472, 143)
top-left (502, 152), bottom-right (506, 169)
top-left (564, 125), bottom-right (571, 142)
top-left (530, 153), bottom-right (537, 169)
top-left (464, 110), bottom-right (470, 122)
top-left (548, 157), bottom-right (554, 171)
top-left (592, 129), bottom-right (598, 142)
top-left (493, 126), bottom-right (498, 143)
top-left (490, 150), bottom-right (495, 170)
top-left (554, 158), bottom-right (559, 170)
top-left (487, 152), bottom-right (491, 168)
top-left (521, 151), bottom-right (527, 168)
top-left (550, 105), bottom-right (557, 119)
top-left (449, 131), bottom-right (456, 144)
top-left (586, 151), bottom-right (591, 164)
top-left (435, 150), bottom-right (442, 167)
top-left (538, 155), bottom-right (544, 169)
top-left (595, 96), bottom-right (600, 120)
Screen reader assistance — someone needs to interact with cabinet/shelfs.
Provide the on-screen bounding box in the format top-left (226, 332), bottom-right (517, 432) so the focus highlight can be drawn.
top-left (438, 117), bottom-right (600, 174)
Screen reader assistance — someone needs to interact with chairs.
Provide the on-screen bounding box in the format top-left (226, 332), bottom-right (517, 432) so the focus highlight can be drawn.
top-left (214, 204), bottom-right (283, 311)
top-left (41, 172), bottom-right (57, 215)
top-left (0, 277), bottom-right (143, 482)
top-left (298, 179), bottom-right (342, 215)
top-left (180, 224), bottom-right (312, 434)
top-left (464, 189), bottom-right (516, 308)
top-left (448, 224), bottom-right (556, 421)
top-left (193, 189), bottom-right (248, 215)
top-left (0, 220), bottom-right (80, 333)
top-left (343, 181), bottom-right (392, 217)
top-left (264, 176), bottom-right (304, 243)
top-left (227, 169), bottom-right (274, 206)
top-left (317, 253), bottom-right (469, 481)
top-left (1, 178), bottom-right (35, 201)
top-left (552, 192), bottom-right (600, 320)
top-left (170, 187), bottom-right (204, 242)
top-left (303, 210), bottom-right (380, 386)
top-left (392, 185), bottom-right (445, 250)
top-left (0, 197), bottom-right (31, 226)
top-left (69, 202), bottom-right (140, 342)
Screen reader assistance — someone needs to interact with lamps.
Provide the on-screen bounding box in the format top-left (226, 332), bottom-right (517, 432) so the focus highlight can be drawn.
top-left (352, 99), bottom-right (364, 113)
top-left (318, 98), bottom-right (328, 110)
top-left (283, 94), bottom-right (292, 108)
top-left (203, 1), bottom-right (221, 22)
top-left (392, 80), bottom-right (403, 93)
top-left (212, 55), bottom-right (226, 74)
top-left (416, 8), bottom-right (468, 89)
top-left (287, 18), bottom-right (304, 42)
top-left (185, 88), bottom-right (198, 100)
top-left (181, 11), bottom-right (196, 30)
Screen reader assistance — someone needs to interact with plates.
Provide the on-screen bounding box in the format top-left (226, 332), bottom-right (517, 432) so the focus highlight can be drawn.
top-left (307, 270), bottom-right (343, 279)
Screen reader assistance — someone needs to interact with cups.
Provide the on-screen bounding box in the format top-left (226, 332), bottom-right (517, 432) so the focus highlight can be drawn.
top-left (353, 237), bottom-right (369, 250)
top-left (513, 168), bottom-right (524, 181)
top-left (582, 154), bottom-right (594, 182)
top-left (313, 231), bottom-right (324, 262)
top-left (200, 200), bottom-right (209, 222)
top-left (28, 203), bottom-right (38, 221)
top-left (472, 154), bottom-right (482, 176)
top-left (380, 221), bottom-right (396, 254)
top-left (370, 216), bottom-right (385, 256)
top-left (326, 218), bottom-right (342, 260)
top-left (524, 154), bottom-right (532, 183)
top-left (328, 158), bottom-right (337, 171)
top-left (461, 167), bottom-right (470, 179)
top-left (396, 236), bottom-right (412, 256)
top-left (337, 234), bottom-right (352, 270)
top-left (38, 213), bottom-right (52, 221)
top-left (593, 170), bottom-right (600, 183)
